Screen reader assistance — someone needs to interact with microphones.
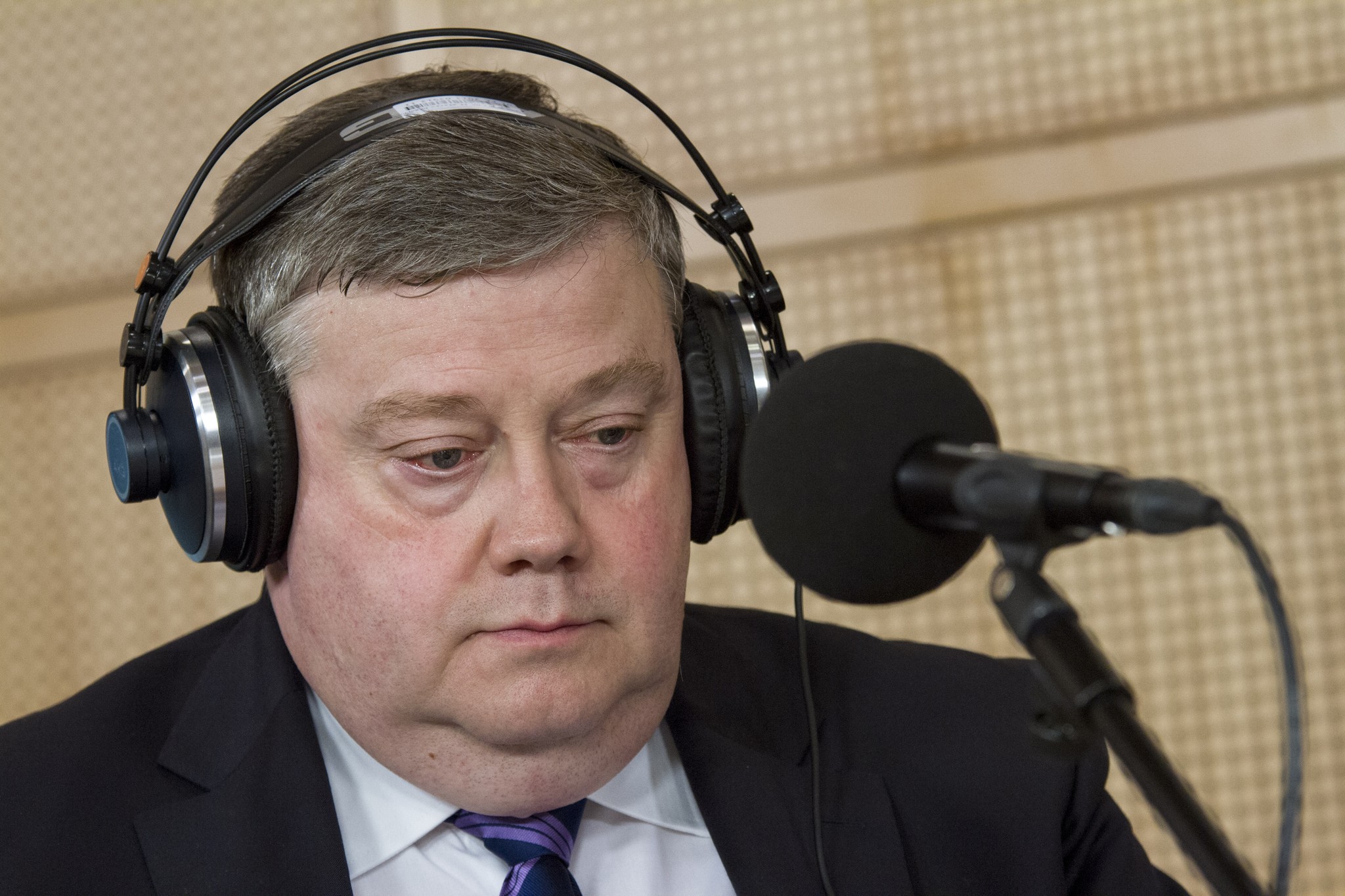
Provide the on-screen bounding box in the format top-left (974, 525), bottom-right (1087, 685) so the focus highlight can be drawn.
top-left (741, 337), bottom-right (1218, 607)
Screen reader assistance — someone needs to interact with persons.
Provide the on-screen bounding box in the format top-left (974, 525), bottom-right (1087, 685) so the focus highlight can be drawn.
top-left (0, 67), bottom-right (1192, 896)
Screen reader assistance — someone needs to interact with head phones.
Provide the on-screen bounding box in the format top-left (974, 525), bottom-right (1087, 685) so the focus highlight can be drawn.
top-left (96, 29), bottom-right (810, 576)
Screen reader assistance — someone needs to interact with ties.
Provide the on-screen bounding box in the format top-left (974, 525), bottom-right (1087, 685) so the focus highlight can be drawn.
top-left (445, 799), bottom-right (588, 896)
